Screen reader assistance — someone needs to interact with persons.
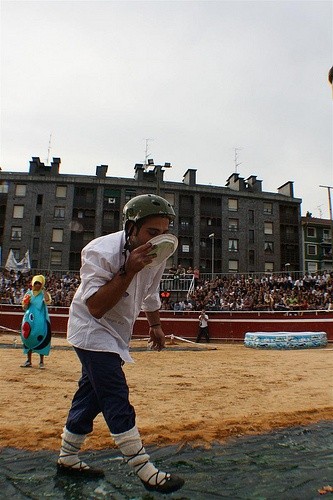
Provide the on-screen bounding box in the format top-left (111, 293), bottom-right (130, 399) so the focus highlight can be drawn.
top-left (196, 309), bottom-right (210, 343)
top-left (159, 291), bottom-right (193, 315)
top-left (35, 272), bottom-right (82, 311)
top-left (20, 275), bottom-right (51, 369)
top-left (166, 264), bottom-right (199, 290)
top-left (188, 270), bottom-right (333, 316)
top-left (56, 194), bottom-right (186, 494)
top-left (0, 268), bottom-right (33, 305)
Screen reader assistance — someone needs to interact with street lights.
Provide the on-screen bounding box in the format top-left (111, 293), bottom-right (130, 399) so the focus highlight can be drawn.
top-left (209, 233), bottom-right (215, 282)
top-left (48, 246), bottom-right (54, 274)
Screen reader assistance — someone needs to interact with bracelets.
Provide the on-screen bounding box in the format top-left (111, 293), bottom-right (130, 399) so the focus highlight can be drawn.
top-left (150, 323), bottom-right (160, 327)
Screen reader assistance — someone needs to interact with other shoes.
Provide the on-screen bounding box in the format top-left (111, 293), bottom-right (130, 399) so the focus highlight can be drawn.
top-left (58, 461), bottom-right (105, 480)
top-left (141, 471), bottom-right (184, 494)
top-left (38, 363), bottom-right (45, 369)
top-left (20, 362), bottom-right (32, 367)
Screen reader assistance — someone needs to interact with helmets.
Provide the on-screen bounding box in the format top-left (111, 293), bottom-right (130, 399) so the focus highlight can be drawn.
top-left (123, 194), bottom-right (177, 231)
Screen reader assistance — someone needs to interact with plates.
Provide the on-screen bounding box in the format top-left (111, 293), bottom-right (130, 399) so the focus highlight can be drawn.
top-left (145, 234), bottom-right (178, 269)
